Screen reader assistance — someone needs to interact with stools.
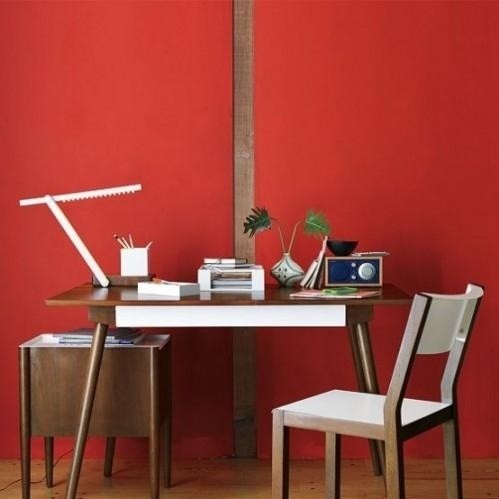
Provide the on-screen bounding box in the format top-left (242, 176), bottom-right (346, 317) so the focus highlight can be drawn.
top-left (16, 331), bottom-right (172, 499)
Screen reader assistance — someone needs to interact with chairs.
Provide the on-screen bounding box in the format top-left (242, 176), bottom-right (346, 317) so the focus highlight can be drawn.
top-left (271, 281), bottom-right (485, 498)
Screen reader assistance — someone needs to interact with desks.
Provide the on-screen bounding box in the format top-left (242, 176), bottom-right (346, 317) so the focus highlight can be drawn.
top-left (42, 285), bottom-right (413, 499)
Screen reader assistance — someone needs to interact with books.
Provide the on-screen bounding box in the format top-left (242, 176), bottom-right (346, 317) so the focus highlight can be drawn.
top-left (289, 287), bottom-right (379, 300)
top-left (203, 255), bottom-right (252, 286)
top-left (53, 327), bottom-right (149, 349)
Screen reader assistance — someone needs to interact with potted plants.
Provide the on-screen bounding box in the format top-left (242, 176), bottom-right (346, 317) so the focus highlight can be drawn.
top-left (241, 206), bottom-right (329, 284)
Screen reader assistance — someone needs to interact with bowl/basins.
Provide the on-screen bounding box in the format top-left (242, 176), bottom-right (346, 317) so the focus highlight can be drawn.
top-left (326, 239), bottom-right (359, 256)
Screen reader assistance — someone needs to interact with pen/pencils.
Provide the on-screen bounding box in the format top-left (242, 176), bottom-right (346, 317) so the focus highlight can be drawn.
top-left (114, 233), bottom-right (153, 248)
